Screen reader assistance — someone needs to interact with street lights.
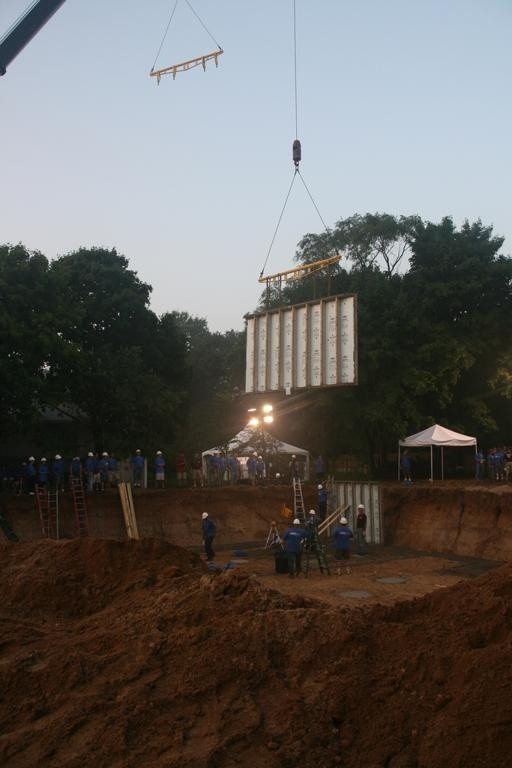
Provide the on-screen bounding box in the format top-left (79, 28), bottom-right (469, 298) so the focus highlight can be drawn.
top-left (247, 403), bottom-right (274, 482)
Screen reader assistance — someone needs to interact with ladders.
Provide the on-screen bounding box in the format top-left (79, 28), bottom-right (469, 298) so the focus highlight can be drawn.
top-left (318, 504), bottom-right (350, 535)
top-left (69, 462), bottom-right (90, 535)
top-left (265, 521), bottom-right (286, 553)
top-left (292, 476), bottom-right (307, 524)
top-left (35, 482), bottom-right (58, 539)
top-left (296, 523), bottom-right (332, 579)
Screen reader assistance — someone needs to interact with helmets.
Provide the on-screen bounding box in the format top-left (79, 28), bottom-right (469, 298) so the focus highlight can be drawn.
top-left (293, 518), bottom-right (300, 524)
top-left (156, 450), bottom-right (162, 456)
top-left (202, 512), bottom-right (208, 519)
top-left (318, 485), bottom-right (322, 490)
top-left (28, 455), bottom-right (62, 462)
top-left (309, 510), bottom-right (316, 514)
top-left (275, 473), bottom-right (280, 479)
top-left (358, 504), bottom-right (364, 508)
top-left (252, 452), bottom-right (262, 458)
top-left (340, 517), bottom-right (348, 524)
top-left (88, 452), bottom-right (108, 457)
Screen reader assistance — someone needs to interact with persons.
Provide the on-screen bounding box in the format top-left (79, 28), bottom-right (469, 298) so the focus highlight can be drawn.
top-left (317, 480), bottom-right (328, 522)
top-left (305, 510), bottom-right (321, 545)
top-left (401, 450), bottom-right (412, 482)
top-left (201, 512), bottom-right (216, 561)
top-left (14, 449), bottom-right (166, 496)
top-left (176, 450), bottom-right (336, 488)
top-left (279, 519), bottom-right (310, 579)
top-left (474, 446), bottom-right (512, 481)
top-left (333, 517), bottom-right (354, 576)
top-left (356, 504), bottom-right (367, 552)
